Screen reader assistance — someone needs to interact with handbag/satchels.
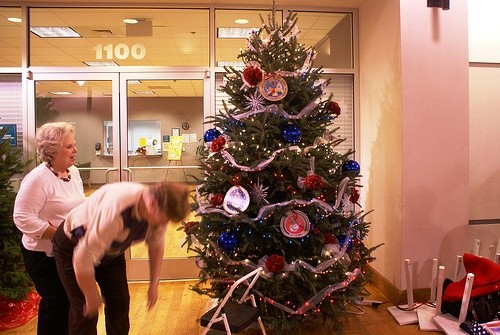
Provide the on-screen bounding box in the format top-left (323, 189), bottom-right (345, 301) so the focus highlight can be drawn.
top-left (441, 277), bottom-right (500, 324)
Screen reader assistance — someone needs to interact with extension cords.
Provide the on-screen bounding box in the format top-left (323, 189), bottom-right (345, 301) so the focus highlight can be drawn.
top-left (356, 299), bottom-right (382, 306)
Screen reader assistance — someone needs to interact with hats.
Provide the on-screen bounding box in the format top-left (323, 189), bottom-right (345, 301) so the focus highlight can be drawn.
top-left (442, 252), bottom-right (500, 301)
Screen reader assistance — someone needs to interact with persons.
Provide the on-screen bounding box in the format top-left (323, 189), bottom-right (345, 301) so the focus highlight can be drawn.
top-left (13, 120), bottom-right (90, 335)
top-left (53, 181), bottom-right (191, 335)
